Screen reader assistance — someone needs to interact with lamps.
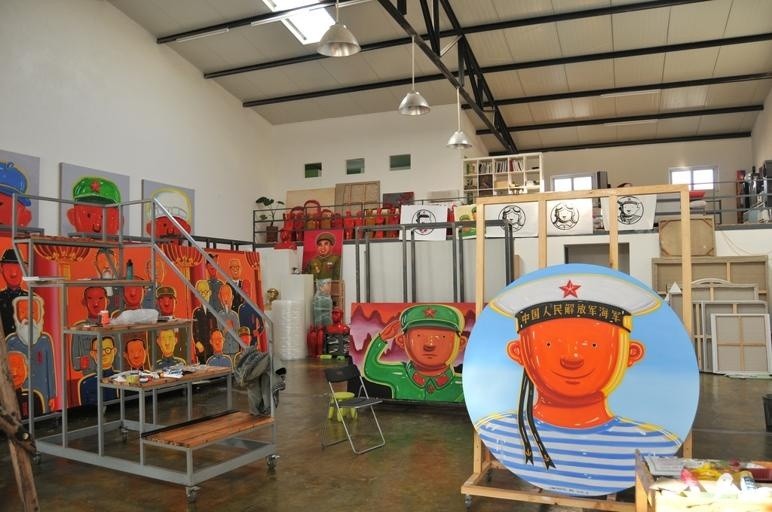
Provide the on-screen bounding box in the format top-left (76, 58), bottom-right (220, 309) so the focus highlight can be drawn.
top-left (398, 36), bottom-right (431, 116)
top-left (446, 88), bottom-right (473, 151)
top-left (316, 1), bottom-right (362, 57)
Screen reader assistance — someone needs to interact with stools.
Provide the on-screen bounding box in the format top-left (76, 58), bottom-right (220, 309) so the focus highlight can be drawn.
top-left (327, 391), bottom-right (358, 422)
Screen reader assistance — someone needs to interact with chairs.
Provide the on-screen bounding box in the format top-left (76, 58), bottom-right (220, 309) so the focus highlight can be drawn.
top-left (321, 365), bottom-right (386, 455)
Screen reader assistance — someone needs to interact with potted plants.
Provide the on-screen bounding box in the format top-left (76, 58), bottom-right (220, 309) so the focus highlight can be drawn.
top-left (256, 196), bottom-right (285, 243)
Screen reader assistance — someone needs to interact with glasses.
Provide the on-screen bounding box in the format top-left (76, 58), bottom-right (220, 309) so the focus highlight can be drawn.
top-left (92, 347), bottom-right (114, 354)
top-left (230, 265), bottom-right (240, 270)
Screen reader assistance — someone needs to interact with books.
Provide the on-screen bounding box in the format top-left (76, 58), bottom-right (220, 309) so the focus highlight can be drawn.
top-left (464, 158), bottom-right (525, 205)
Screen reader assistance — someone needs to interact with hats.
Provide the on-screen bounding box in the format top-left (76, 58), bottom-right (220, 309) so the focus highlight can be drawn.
top-left (157, 286), bottom-right (175, 297)
top-left (0, 161), bottom-right (32, 206)
top-left (317, 233), bottom-right (334, 245)
top-left (238, 325), bottom-right (250, 336)
top-left (617, 196), bottom-right (641, 205)
top-left (399, 305), bottom-right (461, 333)
top-left (472, 207), bottom-right (476, 213)
top-left (148, 191), bottom-right (190, 221)
top-left (0, 248), bottom-right (27, 263)
top-left (460, 215), bottom-right (470, 221)
top-left (490, 273), bottom-right (661, 333)
top-left (73, 177), bottom-right (121, 207)
top-left (206, 253), bottom-right (217, 264)
top-left (417, 211), bottom-right (430, 218)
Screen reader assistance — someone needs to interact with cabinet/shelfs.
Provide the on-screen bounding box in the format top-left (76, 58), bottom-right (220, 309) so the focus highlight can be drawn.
top-left (461, 152), bottom-right (543, 204)
